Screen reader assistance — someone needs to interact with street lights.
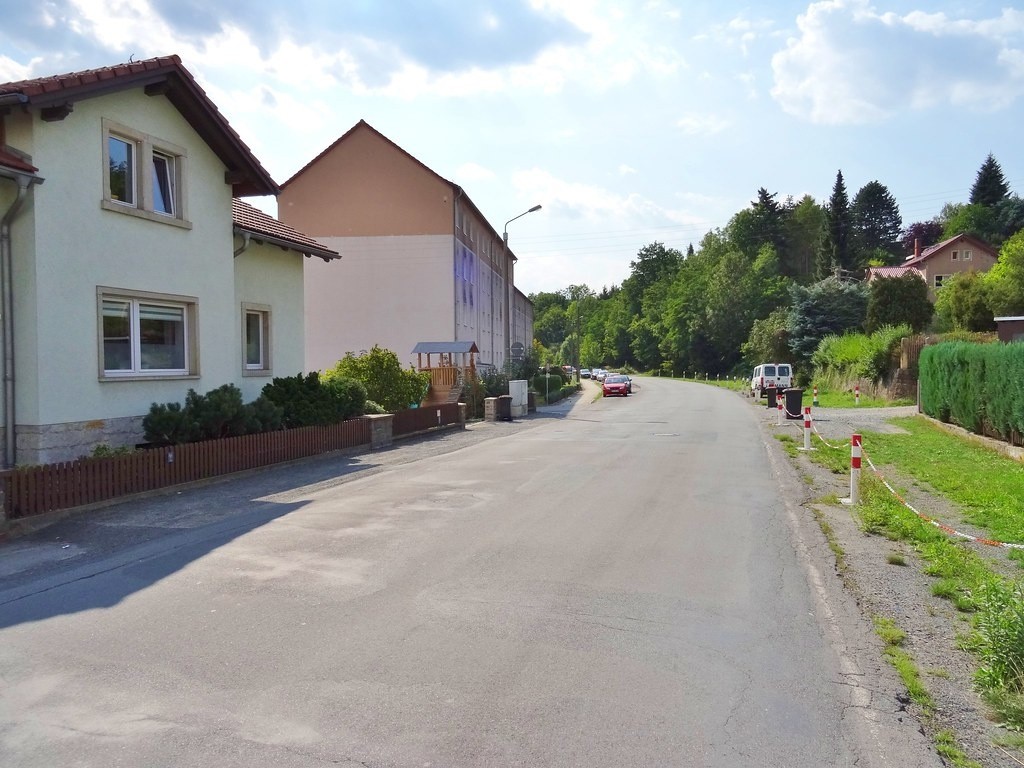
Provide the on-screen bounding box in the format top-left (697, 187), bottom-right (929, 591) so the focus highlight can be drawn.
top-left (501, 204), bottom-right (542, 378)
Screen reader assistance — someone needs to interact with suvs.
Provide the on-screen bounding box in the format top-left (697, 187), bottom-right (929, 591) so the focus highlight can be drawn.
top-left (591, 369), bottom-right (602, 380)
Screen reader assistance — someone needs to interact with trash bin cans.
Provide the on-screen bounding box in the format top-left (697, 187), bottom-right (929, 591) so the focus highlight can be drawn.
top-left (782, 388), bottom-right (806, 419)
top-left (765, 386), bottom-right (779, 407)
top-left (499, 396), bottom-right (513, 421)
top-left (528, 393), bottom-right (536, 412)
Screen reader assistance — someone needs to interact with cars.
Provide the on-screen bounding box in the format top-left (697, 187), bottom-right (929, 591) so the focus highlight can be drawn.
top-left (612, 375), bottom-right (632, 393)
top-left (601, 376), bottom-right (627, 397)
top-left (603, 373), bottom-right (620, 378)
top-left (597, 370), bottom-right (608, 382)
top-left (579, 368), bottom-right (591, 378)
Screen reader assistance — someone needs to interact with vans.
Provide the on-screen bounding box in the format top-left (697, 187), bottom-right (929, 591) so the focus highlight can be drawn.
top-left (748, 364), bottom-right (794, 398)
top-left (562, 365), bottom-right (577, 376)
